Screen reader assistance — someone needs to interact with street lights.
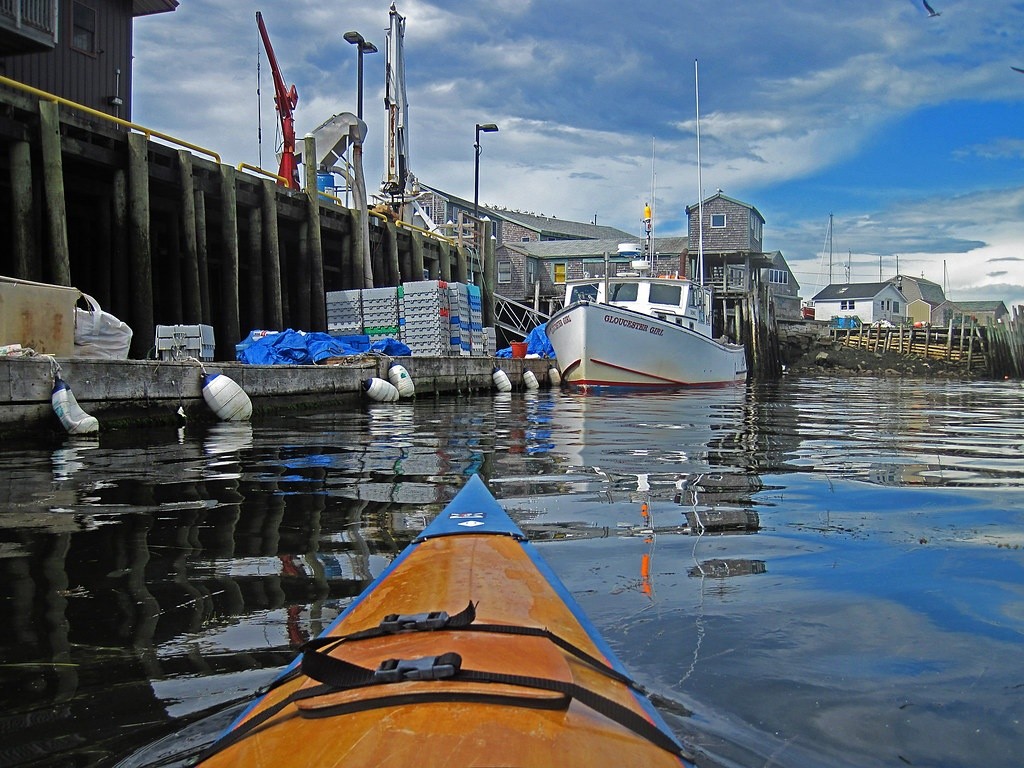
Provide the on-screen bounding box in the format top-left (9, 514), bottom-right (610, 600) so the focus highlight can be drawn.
top-left (344, 31), bottom-right (379, 120)
top-left (473, 123), bottom-right (499, 250)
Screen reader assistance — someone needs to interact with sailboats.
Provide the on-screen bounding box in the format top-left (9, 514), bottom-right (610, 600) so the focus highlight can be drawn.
top-left (545, 55), bottom-right (747, 395)
top-left (802, 212), bottom-right (848, 320)
